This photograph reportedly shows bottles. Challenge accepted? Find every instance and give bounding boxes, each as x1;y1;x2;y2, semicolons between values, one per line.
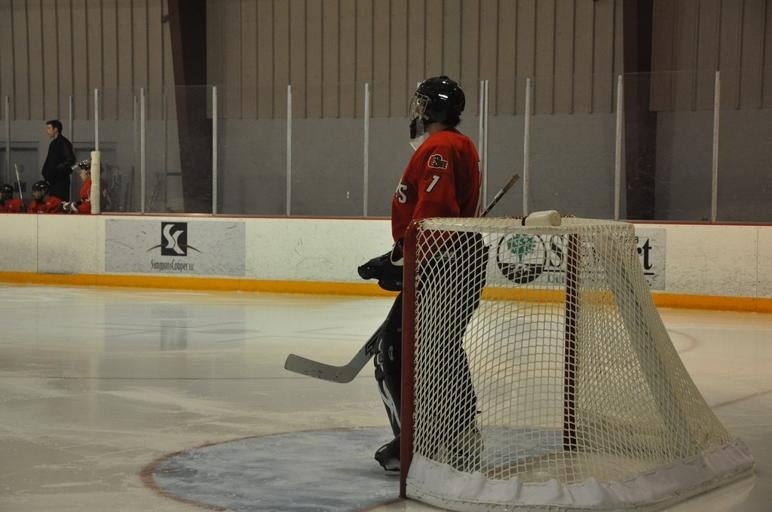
522;210;561;226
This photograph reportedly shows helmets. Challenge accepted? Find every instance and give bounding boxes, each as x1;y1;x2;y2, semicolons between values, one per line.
0;184;14;194
31;181;50;204
78;158;104;175
408;75;465;139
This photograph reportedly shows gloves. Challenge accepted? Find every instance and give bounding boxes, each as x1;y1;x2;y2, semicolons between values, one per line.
61;201;79;212
358;236;404;292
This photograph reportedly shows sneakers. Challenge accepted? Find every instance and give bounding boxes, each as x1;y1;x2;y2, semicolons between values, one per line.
374;430;485;470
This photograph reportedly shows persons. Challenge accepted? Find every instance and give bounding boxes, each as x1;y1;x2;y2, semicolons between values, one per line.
40;119;76;201
0;184;23;213
26;180;65;214
354;74;491;475
58;159;113;215
